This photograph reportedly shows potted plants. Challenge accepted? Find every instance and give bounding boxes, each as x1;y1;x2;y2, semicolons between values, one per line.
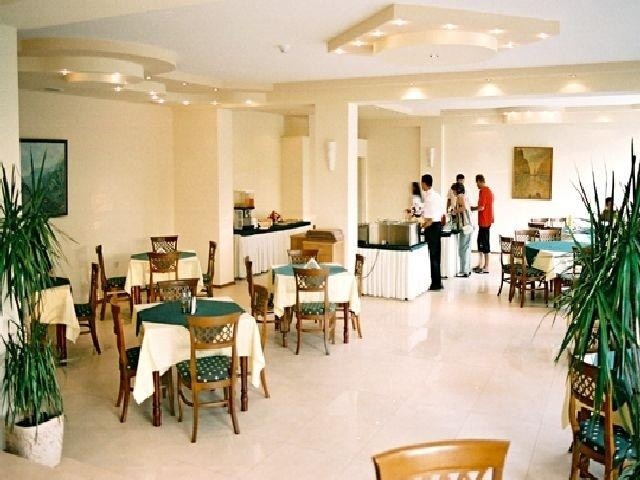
0;159;77;468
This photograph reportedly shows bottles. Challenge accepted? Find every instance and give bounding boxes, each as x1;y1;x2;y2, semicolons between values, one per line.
271;210;278;224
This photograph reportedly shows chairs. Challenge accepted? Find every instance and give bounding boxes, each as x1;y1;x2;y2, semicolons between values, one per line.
573;318;621;353
568;348;639;480
498;218;575;309
55;261;102;357
371;439;510;480
95;234;221;320
107;276;272;444
244;247;366;356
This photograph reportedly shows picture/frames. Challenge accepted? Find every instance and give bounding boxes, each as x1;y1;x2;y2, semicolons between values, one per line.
512;147;553;200
19;138;69;218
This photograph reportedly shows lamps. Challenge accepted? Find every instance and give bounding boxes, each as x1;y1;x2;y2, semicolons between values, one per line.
429;146;434;168
327;4;559;73
19;39;177;104
326;141;335;171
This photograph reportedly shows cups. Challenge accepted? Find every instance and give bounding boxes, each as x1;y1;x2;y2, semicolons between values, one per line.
180;289;197;316
251;217;256;229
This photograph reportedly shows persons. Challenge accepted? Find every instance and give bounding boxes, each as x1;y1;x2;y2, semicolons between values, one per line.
405;181;423;219
456;174;465;184
419;173;447;292
450;182;472;278
470;174;494;273
599;197;618;220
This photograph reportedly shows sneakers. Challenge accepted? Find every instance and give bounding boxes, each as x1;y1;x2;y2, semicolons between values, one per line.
454;265;489;278
